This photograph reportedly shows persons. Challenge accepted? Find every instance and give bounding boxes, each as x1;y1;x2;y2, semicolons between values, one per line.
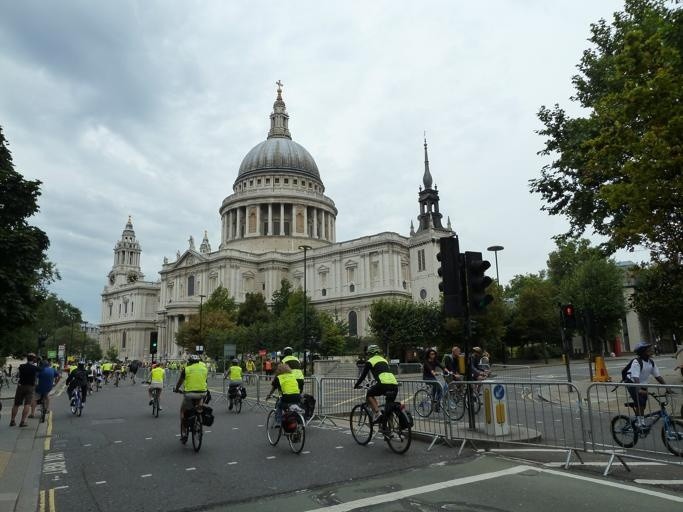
265;359;273;380
423;347;490;410
9;353;218;440
223;359;243;409
246;358;256;374
624;342;672;432
267;365;301;442
280;347;304;394
354;345;398;441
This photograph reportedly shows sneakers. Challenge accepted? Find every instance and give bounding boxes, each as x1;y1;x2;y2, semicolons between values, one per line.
635;420;648;431
371;410;383;424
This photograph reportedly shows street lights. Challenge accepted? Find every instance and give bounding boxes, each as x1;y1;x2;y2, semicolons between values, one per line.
198;294;207;343
297;244;313;376
53;300;61;347
81;320;88;332
68;312;79;353
308;334;317;361
487;245;504;287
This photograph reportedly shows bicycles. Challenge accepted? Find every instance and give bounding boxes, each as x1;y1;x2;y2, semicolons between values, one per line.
263;394;307;455
65;384;84;417
39;393;48;423
609;388;683;458
142;381;162;418
348;378;415;456
0;358;129;395
221;376;243;414
412;370;488;422
171;388;205;452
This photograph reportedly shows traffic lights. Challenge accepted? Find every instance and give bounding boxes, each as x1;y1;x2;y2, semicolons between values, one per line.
462;249;493;319
434;234;465;318
150;331;158;355
560;304;577;335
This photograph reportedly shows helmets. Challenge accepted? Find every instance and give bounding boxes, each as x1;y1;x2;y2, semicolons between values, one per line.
367;345;381;354
78;361;86;365
232;359;238;363
188;355;200;361
28;353;37;357
473;347;482;354
634;342;651;353
